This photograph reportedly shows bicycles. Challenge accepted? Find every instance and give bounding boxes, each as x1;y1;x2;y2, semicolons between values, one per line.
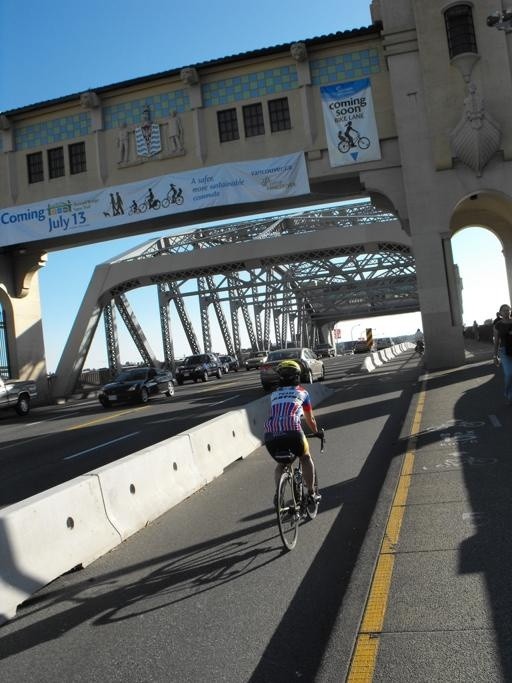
138;195;161;213
162;194;184;208
275;427;326;551
338;131;371;153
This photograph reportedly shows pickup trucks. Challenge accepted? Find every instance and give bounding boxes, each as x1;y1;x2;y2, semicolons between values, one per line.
0;374;38;416
313;343;335;358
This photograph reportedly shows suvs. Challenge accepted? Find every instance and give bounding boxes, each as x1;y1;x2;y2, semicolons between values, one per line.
175;353;222;385
376;336;394;350
354;340;375;353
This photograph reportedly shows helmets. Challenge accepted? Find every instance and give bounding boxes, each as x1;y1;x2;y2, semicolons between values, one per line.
276;360;300;375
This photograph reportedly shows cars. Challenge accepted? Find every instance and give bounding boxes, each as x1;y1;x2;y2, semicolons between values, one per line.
344;349;354;355
260;347;325;392
104;365;137;385
218;356;238;373
245;350;271;371
98;367;178;408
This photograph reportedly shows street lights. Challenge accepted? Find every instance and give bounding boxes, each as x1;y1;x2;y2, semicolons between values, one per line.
351;323;360;340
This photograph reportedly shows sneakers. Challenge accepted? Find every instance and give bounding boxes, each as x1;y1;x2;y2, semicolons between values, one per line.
307;494;322;503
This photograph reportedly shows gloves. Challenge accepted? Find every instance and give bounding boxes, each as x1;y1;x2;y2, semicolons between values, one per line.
316;428;325;437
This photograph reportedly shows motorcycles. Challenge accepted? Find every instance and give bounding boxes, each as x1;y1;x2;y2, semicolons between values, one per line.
416;339;424;355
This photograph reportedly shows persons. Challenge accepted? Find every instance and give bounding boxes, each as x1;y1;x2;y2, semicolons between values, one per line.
158;108;183;151
415;328;424;348
264;361;322;509
491;304;512;401
115;120;135;164
473;320;480;341
